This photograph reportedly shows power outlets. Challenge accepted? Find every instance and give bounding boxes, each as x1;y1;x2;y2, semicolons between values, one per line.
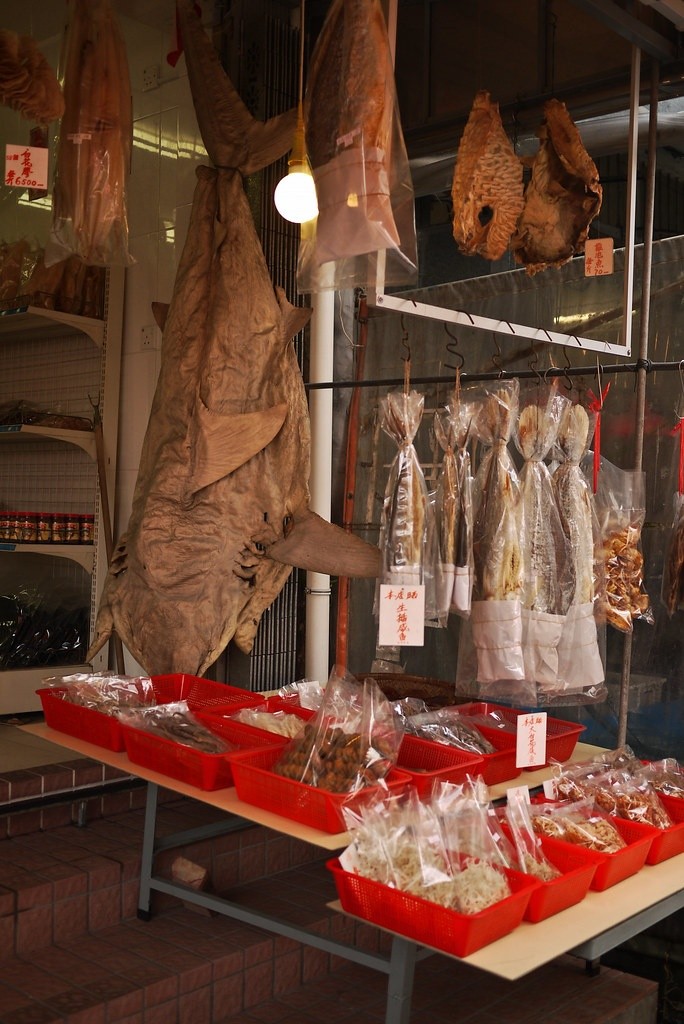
141;326;157;350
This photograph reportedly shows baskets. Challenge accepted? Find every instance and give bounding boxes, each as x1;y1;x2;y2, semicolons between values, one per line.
545;670;666;724
326;760;684;957
35;672;586;834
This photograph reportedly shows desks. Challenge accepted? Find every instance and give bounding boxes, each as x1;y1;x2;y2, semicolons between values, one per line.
16;690;684;1024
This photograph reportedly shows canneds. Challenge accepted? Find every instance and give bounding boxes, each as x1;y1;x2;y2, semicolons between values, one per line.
0;510;94;543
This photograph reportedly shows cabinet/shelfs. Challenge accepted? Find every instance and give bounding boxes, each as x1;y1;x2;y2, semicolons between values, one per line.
0;259;124;716
